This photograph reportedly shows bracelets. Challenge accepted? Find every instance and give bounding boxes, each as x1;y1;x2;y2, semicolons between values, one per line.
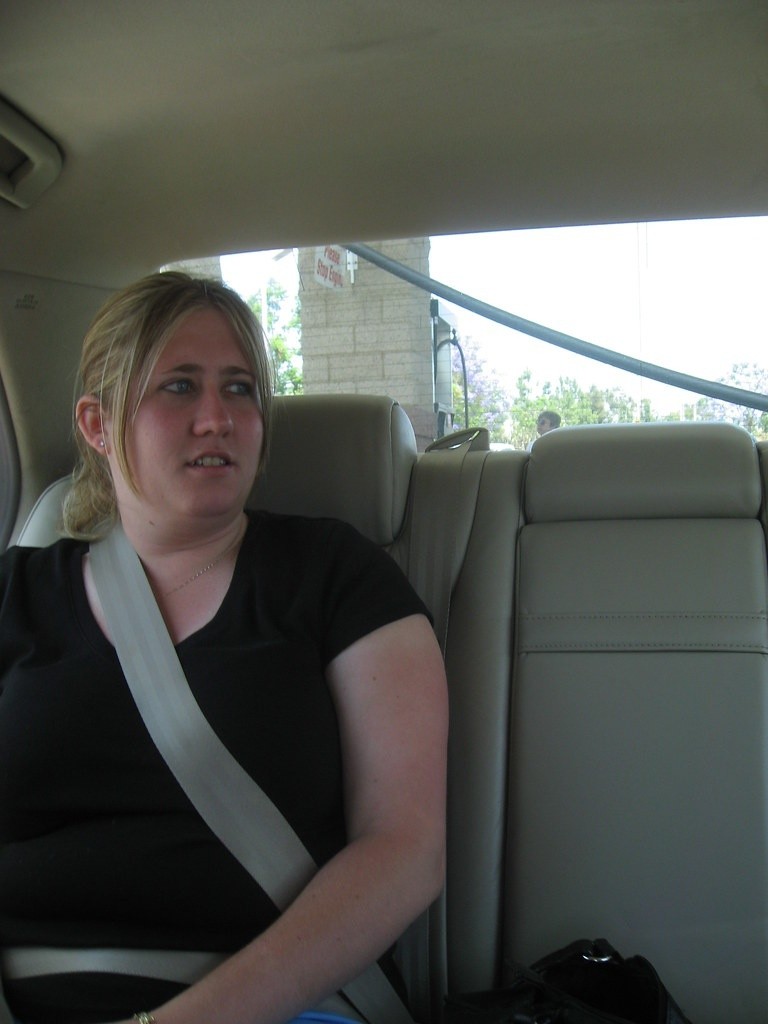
134;1012;158;1024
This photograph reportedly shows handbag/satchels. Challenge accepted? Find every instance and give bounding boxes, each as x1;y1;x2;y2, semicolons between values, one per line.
444;938;689;1024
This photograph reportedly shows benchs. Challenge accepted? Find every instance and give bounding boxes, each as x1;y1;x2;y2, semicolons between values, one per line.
8;388;768;1024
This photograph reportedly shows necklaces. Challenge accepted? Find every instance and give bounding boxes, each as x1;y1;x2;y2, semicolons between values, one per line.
157;521;243;599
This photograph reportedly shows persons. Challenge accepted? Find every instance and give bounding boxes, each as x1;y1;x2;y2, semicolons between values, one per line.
1;268;449;1024
537;411;560;435
